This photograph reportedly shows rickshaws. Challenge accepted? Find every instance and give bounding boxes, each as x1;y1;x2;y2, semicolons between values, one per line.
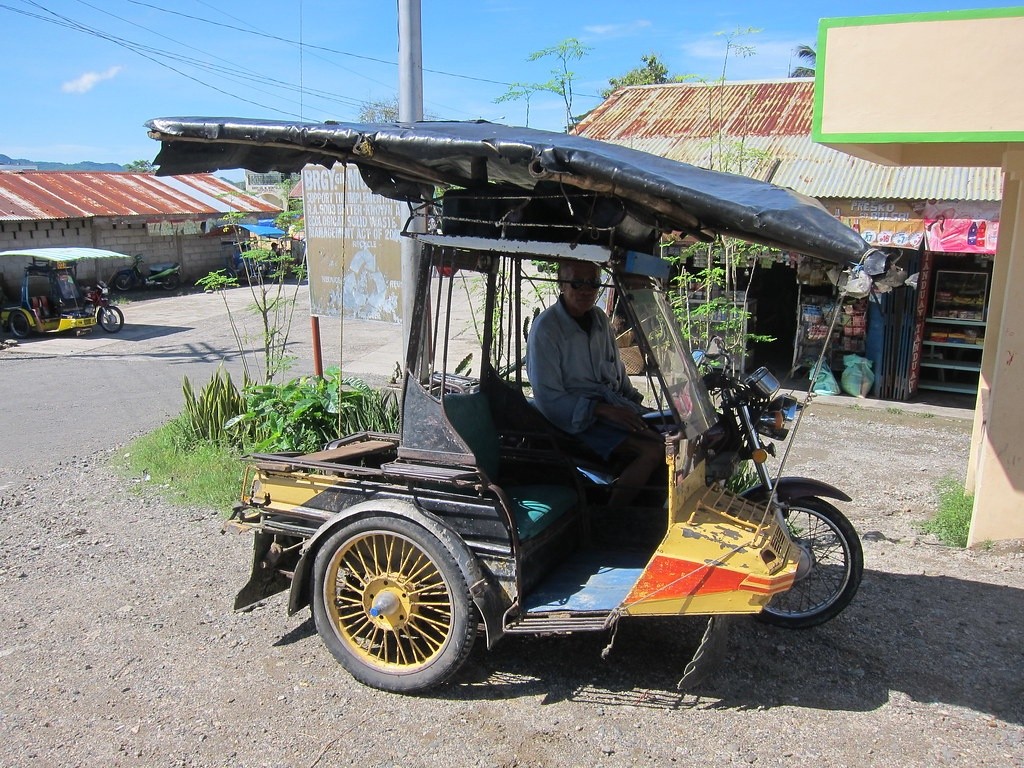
140;115;895;697
0;247;136;339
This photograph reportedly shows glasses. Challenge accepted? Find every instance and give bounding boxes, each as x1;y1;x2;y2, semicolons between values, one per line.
560;277;601;290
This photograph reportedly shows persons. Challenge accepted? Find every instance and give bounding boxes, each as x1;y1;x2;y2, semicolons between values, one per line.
269;243;280;264
234;245;251;276
526;257;666;503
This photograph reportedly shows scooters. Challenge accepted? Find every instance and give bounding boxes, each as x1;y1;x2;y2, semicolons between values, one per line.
112;254;183;292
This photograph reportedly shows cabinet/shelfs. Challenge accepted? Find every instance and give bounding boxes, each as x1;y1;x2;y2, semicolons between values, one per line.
917;319;987;396
689;296;758;374
932;270;989;322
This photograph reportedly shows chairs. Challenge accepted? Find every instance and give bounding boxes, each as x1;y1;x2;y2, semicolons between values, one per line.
417;392;596;584
29;295;54;319
480;355;677;517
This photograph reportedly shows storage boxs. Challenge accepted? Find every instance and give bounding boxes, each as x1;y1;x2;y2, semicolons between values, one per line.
844;311;868;337
